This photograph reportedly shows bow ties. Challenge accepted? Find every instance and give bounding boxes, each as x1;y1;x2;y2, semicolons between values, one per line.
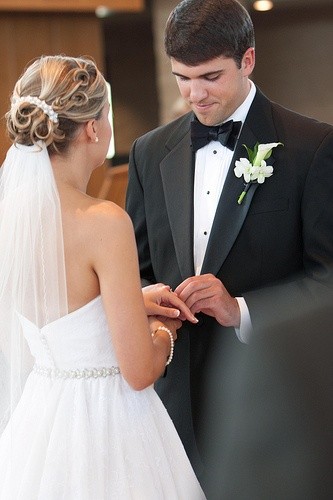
191;120;242;151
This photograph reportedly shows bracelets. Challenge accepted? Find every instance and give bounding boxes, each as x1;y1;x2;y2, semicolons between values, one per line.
152;326;174;367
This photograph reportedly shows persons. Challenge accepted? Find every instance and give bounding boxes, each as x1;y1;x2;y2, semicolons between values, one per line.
0;55;207;500
126;0;332;499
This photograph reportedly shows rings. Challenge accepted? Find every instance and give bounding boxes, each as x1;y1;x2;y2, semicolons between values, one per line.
170;287;172;293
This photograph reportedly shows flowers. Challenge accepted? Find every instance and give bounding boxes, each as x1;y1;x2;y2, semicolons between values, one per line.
234;142;284;205
